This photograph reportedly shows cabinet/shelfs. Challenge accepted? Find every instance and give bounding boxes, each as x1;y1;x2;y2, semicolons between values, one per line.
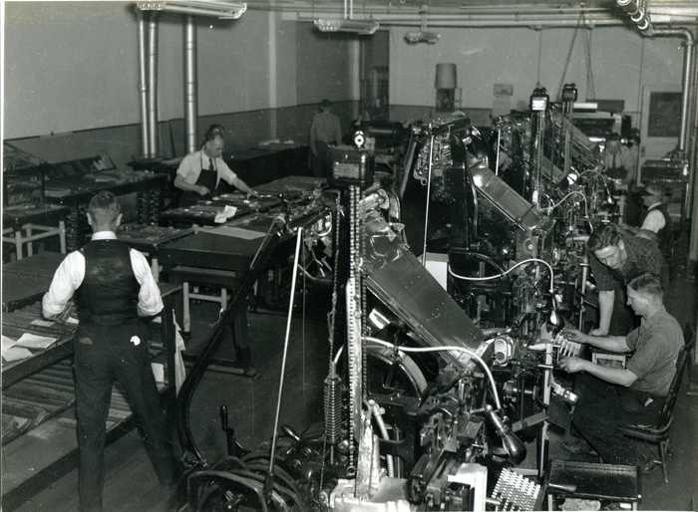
2;138;334;512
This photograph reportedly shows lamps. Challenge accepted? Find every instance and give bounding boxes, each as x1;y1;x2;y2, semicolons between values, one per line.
404;11;441;44
137;0;247;20
313;0;380;35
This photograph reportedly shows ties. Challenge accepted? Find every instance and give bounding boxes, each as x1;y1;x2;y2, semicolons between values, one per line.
209;159;213;169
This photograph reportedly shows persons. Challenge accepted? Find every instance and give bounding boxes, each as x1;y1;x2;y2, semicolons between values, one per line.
42;188;183;511
584;222;667;341
175;122;262;199
307;96;342;180
601;132;635;187
558;268;685;475
640;179;671;248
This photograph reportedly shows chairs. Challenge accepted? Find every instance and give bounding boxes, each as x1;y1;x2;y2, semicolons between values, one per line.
614;323;697;485
